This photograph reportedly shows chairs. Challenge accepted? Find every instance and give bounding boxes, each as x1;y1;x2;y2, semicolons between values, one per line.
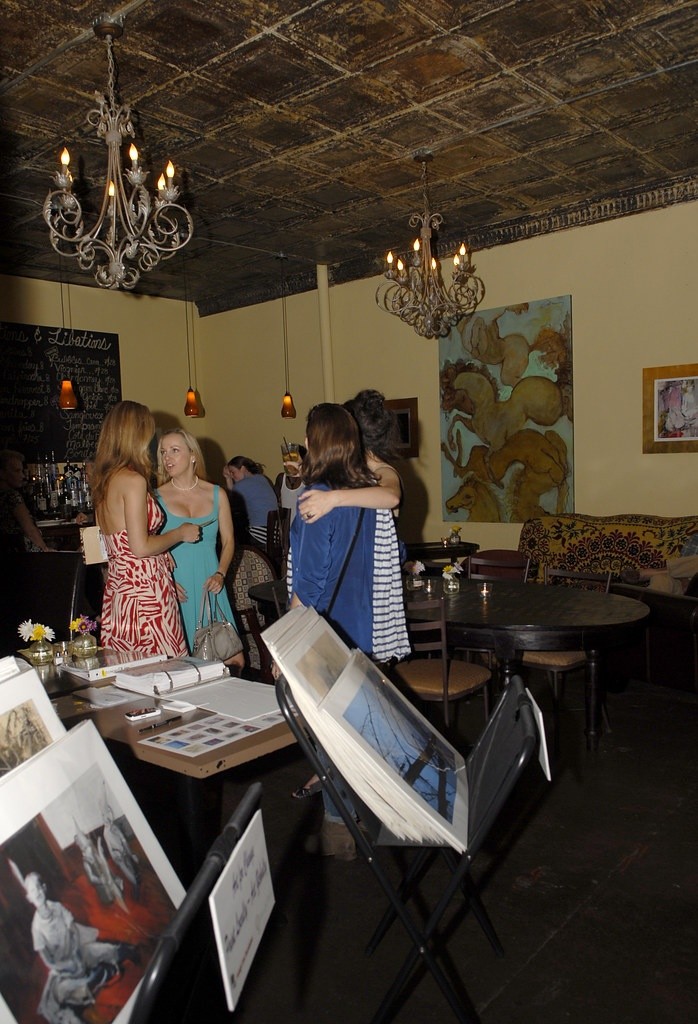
1;505;614;1024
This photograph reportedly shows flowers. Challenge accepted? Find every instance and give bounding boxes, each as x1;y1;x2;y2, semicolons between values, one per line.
68;613;103;633
15;616;59;646
403;560;425;575
441;562;464;579
450;524;462;535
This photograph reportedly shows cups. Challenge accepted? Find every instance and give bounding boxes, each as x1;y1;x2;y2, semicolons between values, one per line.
441;537;450;545
281;443;298;476
422;579;437;593
478;582;494;598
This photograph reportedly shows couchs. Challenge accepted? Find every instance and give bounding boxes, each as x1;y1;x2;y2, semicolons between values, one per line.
519;511;698;684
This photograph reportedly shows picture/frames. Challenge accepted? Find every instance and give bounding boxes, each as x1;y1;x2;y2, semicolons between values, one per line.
643;366;698;454
379;398;418;460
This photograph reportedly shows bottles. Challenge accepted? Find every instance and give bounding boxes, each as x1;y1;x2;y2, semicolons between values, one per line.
22;450;96;520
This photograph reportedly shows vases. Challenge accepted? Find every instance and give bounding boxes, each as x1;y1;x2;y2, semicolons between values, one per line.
443;574;459;594
29;639;54;665
68;633;103;658
406;574;423;590
449;534;459;544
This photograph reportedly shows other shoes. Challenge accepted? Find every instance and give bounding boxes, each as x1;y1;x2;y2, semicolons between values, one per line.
290;780;322;799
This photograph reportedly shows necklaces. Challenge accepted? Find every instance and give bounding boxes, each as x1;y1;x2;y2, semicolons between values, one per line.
171;474;198;491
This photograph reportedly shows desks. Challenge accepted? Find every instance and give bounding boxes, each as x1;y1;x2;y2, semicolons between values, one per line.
245;576;652;761
405;542;479;578
2;644;302;885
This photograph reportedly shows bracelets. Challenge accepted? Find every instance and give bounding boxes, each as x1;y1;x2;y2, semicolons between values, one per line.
216;571;224;579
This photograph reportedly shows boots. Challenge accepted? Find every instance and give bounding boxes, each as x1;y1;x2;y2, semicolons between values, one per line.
300;818;369;861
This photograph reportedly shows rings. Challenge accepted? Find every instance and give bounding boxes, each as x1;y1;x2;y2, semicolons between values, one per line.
307;513;311;518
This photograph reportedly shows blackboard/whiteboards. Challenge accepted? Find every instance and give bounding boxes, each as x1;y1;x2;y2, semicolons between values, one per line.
0;321;122;463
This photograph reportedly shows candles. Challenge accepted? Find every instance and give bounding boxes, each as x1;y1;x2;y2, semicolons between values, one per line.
443;541;447;545
426;585;432;593
481;590;490;595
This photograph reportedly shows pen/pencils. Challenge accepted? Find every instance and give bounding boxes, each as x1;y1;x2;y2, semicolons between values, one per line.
138;715;182;733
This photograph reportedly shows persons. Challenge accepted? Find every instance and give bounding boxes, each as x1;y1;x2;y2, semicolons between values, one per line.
0;450;59;552
90;388;418;859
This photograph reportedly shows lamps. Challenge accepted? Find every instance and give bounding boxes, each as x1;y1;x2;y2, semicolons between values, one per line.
373;149;486;338
55;232;76;413
42;12;192;291
277;252;298;421
172;232;205;419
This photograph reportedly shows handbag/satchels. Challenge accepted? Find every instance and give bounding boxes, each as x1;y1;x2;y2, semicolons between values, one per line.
192;584;243;662
